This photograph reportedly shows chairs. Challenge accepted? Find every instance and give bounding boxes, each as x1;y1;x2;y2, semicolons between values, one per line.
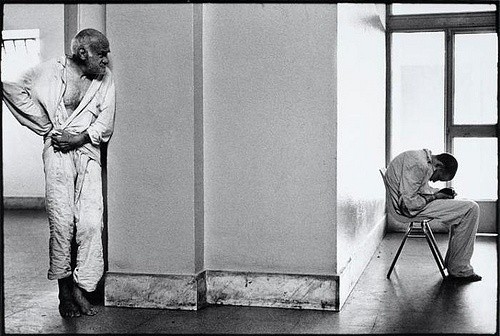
380;166;450;279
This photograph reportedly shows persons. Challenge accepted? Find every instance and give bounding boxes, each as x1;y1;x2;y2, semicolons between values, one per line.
0;29;116;317
385;149;482;281
